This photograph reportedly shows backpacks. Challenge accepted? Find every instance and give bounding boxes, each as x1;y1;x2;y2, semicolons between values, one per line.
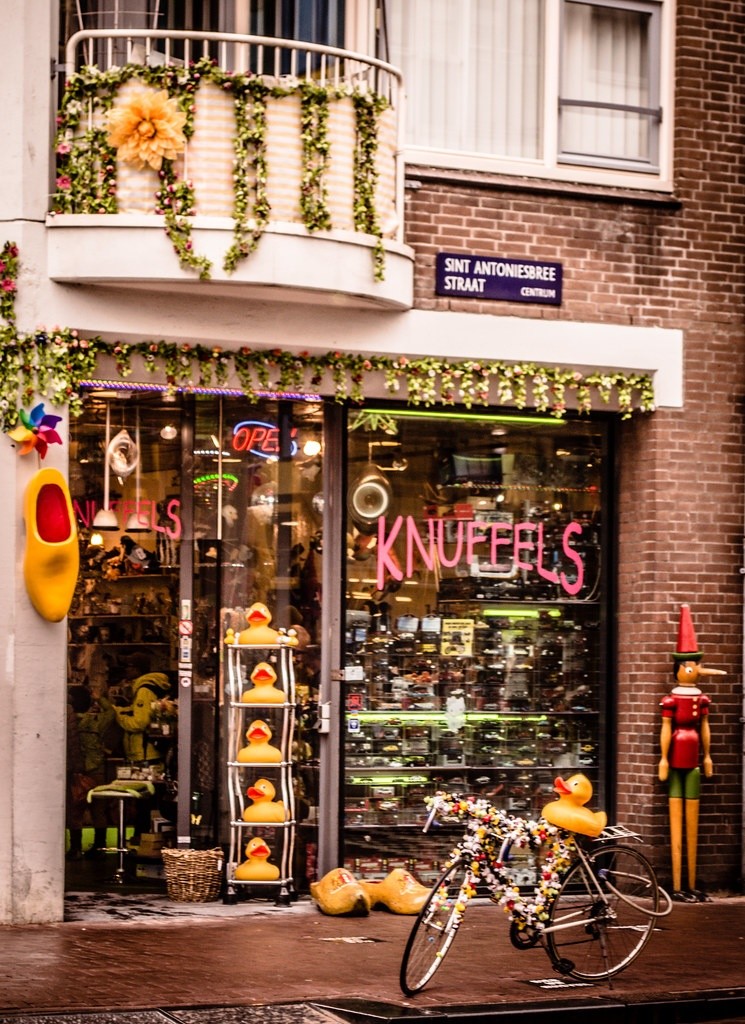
132;684;176;755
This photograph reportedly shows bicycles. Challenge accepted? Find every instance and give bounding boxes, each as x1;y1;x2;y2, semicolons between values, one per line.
399;795;663;996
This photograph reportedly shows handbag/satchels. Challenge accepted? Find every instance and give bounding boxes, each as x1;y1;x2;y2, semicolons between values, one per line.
67;768;96;804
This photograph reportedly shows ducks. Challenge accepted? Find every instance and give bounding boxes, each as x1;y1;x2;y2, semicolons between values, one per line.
541;772;607;837
235;601;291;882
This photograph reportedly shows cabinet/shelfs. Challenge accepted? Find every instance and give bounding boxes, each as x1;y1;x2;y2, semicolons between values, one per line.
227;627;301;908
64;574;171;647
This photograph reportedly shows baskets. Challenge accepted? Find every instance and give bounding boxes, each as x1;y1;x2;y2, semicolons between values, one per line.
160;847;223;903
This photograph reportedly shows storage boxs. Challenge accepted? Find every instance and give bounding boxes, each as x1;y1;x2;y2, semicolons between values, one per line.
421;496;602;600
303;617;599;886
118;765;174;857
136;863;167;880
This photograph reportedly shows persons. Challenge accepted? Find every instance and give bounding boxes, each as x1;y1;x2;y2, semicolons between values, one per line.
66;685;118;860
114;652;177;846
658;603;729;903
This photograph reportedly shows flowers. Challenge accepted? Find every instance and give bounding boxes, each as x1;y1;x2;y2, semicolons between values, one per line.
104;77;189;172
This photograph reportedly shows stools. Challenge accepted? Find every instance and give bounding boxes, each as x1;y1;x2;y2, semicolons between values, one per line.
91;790;147;884
